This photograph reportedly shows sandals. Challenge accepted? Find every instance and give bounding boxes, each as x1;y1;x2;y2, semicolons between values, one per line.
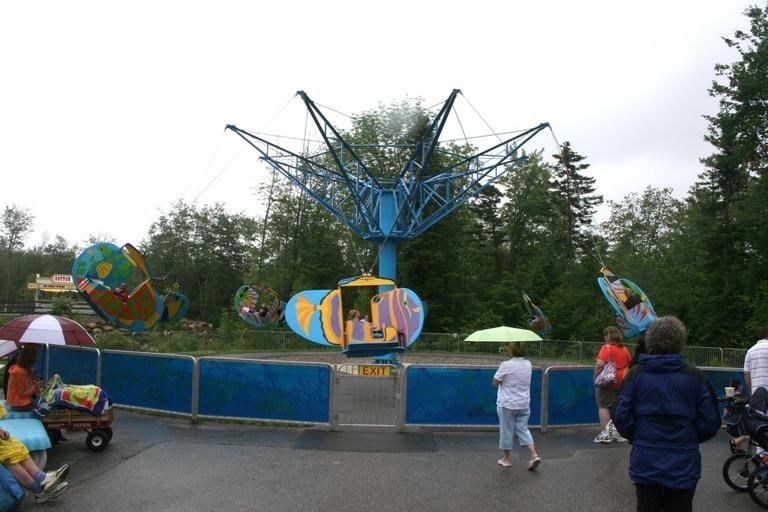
728;439;737;455
734;447;747;454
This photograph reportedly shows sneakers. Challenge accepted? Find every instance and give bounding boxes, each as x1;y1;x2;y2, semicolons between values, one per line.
32;482;68;504
41;464;71;493
526;456;543;472
593;434;612;444
498;457;512;469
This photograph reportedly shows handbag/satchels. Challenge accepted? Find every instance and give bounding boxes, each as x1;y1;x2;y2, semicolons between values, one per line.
592;360;618;387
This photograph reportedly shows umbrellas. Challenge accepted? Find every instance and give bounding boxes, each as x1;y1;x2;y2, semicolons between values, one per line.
464;326;544;362
0;314;96;388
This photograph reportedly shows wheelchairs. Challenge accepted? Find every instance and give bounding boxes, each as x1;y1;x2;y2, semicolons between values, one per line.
717;388;768;512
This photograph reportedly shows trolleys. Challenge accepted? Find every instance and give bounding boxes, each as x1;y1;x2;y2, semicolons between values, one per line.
36;383;113;451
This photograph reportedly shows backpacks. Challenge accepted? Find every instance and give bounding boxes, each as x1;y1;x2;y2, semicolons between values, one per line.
0;461;26;512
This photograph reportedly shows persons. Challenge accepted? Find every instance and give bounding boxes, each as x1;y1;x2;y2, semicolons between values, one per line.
0;403;69;504
244;303;269;321
623;287;642;310
743;326;768;459
347;308;361;321
592;326;632;443
493;343;541;471
722;377;751;454
361;314;369;321
114;282;132;305
7;349;64;445
3;348;69;447
612;315;722;511
